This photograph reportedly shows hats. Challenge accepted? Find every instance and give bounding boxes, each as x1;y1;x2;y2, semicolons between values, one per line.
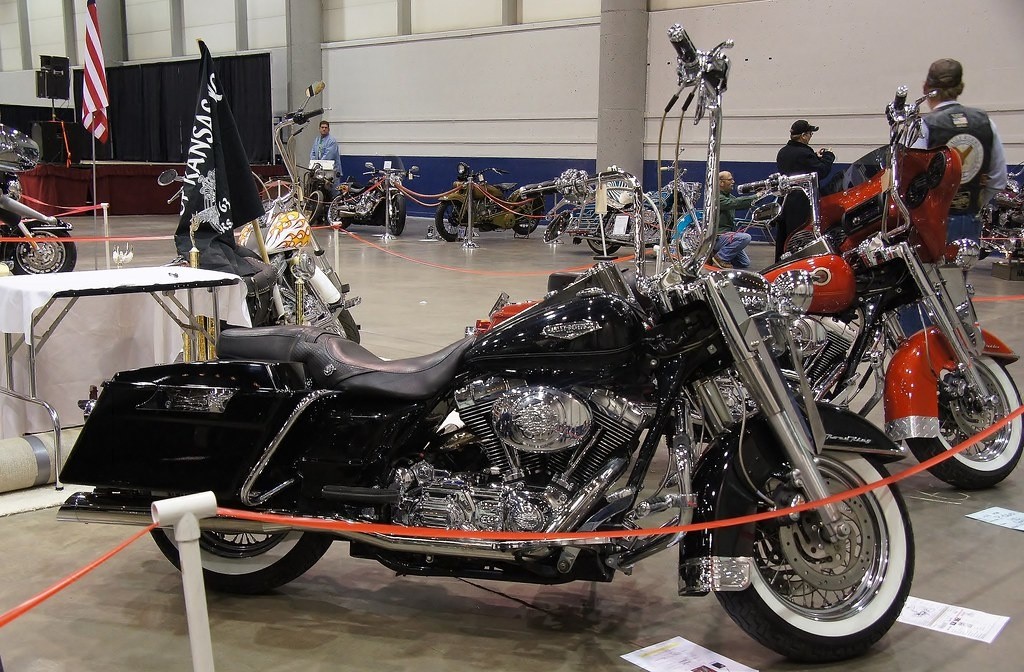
789;120;820;136
928;58;963;88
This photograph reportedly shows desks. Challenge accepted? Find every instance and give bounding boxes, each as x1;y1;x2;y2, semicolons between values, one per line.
0;265;240;490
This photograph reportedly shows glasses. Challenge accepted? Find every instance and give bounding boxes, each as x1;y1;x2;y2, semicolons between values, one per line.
720;178;734;181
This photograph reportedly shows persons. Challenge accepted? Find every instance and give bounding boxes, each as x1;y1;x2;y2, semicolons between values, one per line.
775;120;836;263
897;57;1008;338
310;121;343;226
711;170;765;269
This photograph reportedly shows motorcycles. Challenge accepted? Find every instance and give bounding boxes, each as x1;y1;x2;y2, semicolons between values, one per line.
296;160;1023;278
54;25;918;666
156;79;362;344
465;83;1024;492
0;123;76;277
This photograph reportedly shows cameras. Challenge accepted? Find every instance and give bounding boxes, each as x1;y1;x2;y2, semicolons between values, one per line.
820;149;828;155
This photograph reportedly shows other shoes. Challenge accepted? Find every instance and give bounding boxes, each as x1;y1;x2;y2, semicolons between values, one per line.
705;250;716;266
713;253;733;269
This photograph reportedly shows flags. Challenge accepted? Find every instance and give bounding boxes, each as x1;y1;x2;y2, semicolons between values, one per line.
174;37;266;277
82;0;110;144
